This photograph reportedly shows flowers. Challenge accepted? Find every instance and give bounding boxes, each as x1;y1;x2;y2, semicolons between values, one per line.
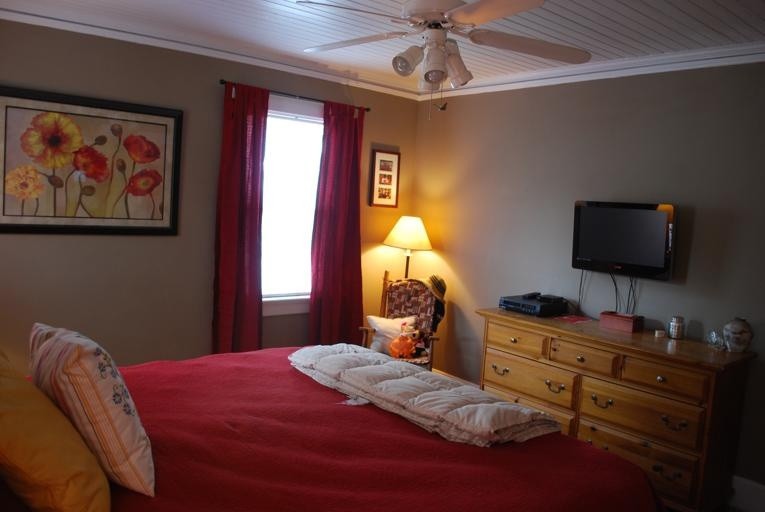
4;106;165;219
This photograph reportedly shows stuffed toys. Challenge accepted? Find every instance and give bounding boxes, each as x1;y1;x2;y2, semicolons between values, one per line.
388;320;427;359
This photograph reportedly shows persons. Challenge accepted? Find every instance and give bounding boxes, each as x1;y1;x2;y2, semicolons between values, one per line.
378;160;392;199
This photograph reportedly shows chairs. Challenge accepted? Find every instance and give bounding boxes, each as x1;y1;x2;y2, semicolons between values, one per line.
357;271;446;371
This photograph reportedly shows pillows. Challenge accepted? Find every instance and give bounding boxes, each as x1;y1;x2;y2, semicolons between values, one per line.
27;322;162;499
0;365;111;512
362;314;417;352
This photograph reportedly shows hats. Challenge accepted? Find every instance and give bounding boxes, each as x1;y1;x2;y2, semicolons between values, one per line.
420;274;447;303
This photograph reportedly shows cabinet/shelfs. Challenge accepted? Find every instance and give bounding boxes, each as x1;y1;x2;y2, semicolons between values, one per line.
471;298;753;509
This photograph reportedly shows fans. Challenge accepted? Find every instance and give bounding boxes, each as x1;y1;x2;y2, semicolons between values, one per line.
283;0;593;94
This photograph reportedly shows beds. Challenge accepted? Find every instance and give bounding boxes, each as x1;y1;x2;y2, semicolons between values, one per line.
0;345;665;511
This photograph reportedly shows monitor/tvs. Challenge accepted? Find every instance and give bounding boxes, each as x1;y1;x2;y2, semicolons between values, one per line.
570;198;677;286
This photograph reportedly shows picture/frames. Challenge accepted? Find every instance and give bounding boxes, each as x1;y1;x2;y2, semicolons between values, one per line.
0;84;187;240
369;148;401;207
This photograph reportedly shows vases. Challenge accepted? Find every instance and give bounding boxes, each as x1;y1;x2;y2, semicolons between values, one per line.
720;314;752;355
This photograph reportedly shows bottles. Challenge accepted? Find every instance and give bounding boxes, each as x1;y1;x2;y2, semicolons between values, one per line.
669;316;685;339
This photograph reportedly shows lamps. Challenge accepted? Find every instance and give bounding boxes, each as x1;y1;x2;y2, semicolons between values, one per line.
386;32;473;116
377;215;433;279
